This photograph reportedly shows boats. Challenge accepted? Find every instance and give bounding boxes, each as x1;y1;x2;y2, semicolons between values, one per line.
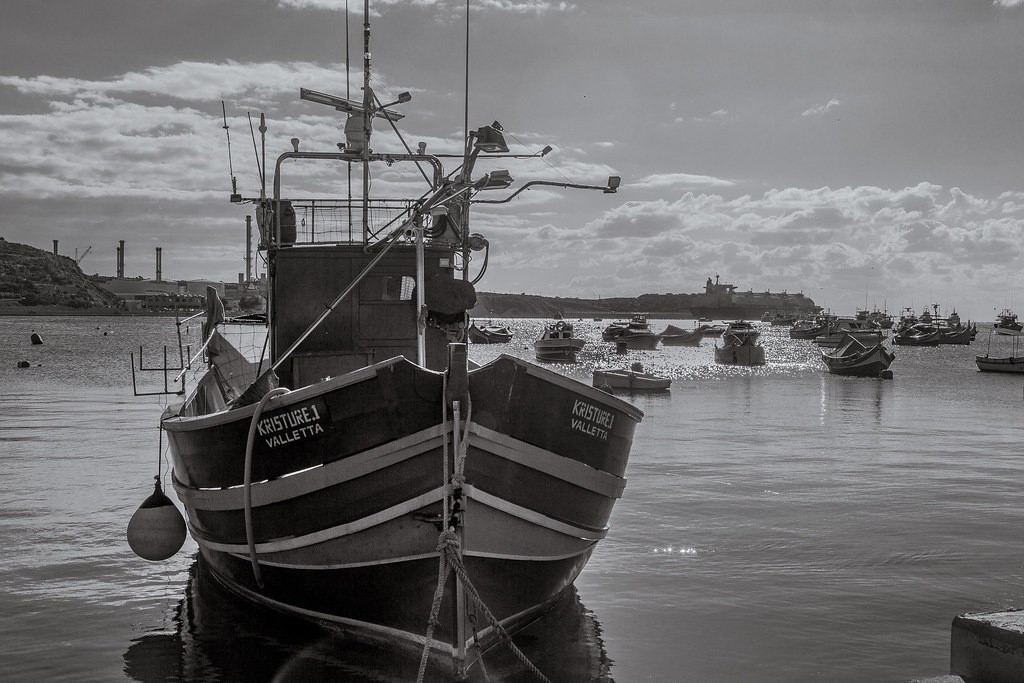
125;1;645;678
976;330;1024;374
721;322;761;348
757;302;1024;348
657;324;707;345
818;334;896;374
697;320;730;336
713;330;766;366
590;363;671;391
616;314;661;351
123;557;613;683
